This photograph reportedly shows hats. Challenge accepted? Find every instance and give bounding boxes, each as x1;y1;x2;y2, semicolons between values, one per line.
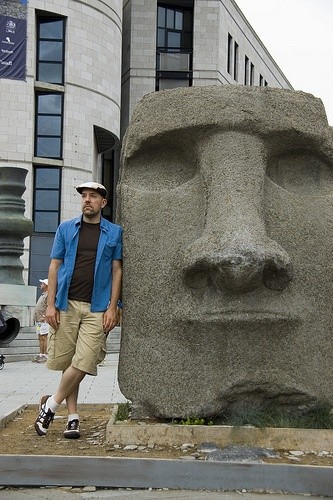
75;182;107;199
38;278;48;285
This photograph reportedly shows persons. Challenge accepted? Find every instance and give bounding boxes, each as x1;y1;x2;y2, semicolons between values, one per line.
33;279;48;363
116;84;331;424
116;293;122;326
34;182;123;438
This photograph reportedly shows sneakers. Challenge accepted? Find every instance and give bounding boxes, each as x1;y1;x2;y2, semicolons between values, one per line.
63;418;81;439
34;394;56;436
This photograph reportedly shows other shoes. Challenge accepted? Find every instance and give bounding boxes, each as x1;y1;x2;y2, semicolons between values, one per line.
31;354;40;362
37;354;47;363
